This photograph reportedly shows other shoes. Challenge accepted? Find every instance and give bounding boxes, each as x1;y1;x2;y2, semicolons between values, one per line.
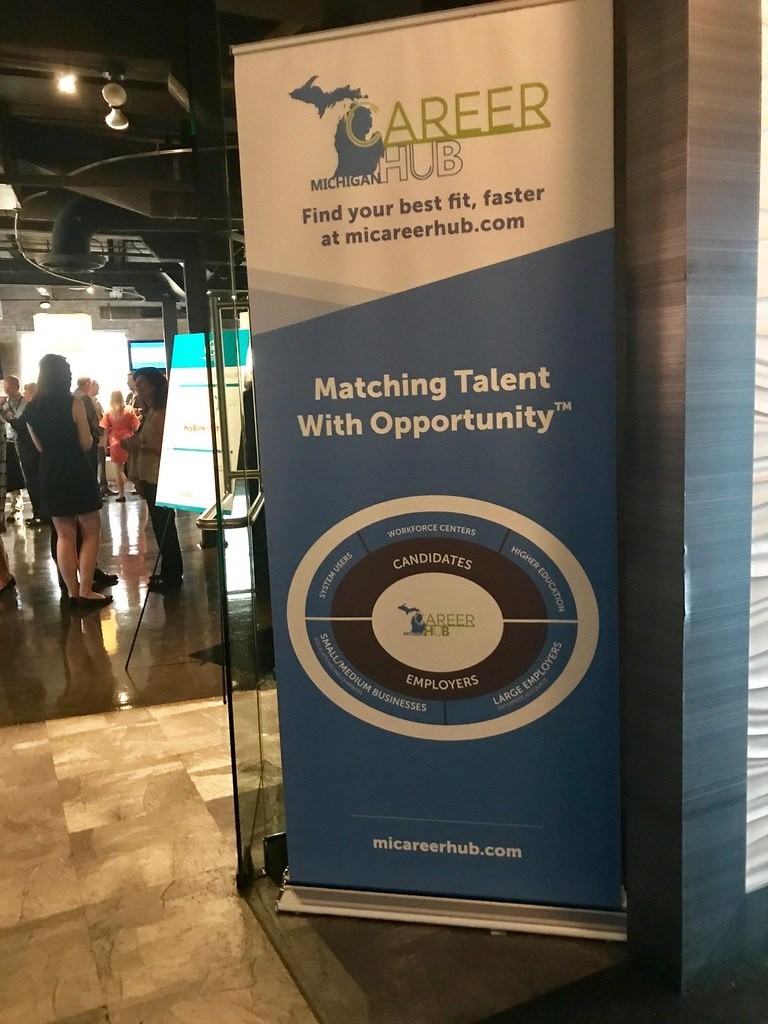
116;497;125;502
148;578;183;589
25;514;50;525
93;569;119;582
0;574;16;596
149;573;164;580
68;597;78;608
6;516;15;522
99;485;118;496
78;592;112;608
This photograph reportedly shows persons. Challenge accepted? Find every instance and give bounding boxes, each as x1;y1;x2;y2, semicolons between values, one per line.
0;354;148;608
120;367;184;589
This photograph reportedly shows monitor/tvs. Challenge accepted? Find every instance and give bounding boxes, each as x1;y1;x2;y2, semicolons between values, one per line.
127;339;166;372
